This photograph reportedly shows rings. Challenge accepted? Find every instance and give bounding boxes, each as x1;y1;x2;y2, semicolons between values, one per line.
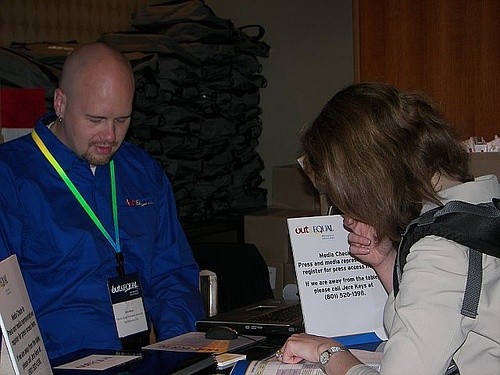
275;350;283;358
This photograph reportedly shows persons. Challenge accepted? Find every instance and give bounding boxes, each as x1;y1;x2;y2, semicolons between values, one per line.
275;82;500;375
0;42;208;361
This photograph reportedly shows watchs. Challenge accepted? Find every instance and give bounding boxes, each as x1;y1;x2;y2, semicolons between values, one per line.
319;347;351;369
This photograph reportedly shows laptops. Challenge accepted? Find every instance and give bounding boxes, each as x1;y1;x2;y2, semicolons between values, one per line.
195;299;305;334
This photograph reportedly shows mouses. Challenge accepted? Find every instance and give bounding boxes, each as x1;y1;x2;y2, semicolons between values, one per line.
205;326;239;340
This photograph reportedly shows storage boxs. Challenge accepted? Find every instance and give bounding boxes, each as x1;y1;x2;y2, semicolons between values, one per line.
244;165;320;300
